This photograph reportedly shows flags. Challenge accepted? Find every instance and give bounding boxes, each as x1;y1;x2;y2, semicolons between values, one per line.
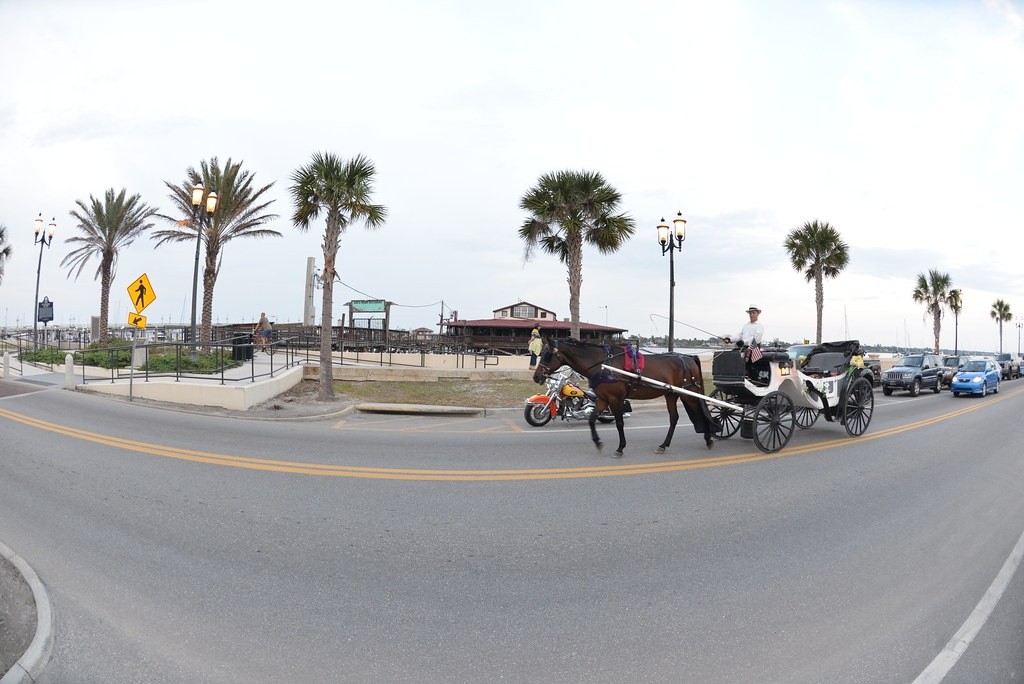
748;339;763;364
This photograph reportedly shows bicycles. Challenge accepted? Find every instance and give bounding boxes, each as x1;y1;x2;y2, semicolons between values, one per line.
250;329;278;356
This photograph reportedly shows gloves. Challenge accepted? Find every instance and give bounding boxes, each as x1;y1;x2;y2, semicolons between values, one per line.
723;337;730;344
736;340;744;347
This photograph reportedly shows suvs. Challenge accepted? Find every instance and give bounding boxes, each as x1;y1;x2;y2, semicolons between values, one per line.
783;341;819;368
942;354;1024;390
881;353;945;398
995;353;1020;379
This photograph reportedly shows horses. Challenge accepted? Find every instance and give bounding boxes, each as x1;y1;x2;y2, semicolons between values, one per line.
533;334;723;460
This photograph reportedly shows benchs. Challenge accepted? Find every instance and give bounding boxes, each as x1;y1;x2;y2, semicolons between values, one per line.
802;353;847;377
732;349;789;363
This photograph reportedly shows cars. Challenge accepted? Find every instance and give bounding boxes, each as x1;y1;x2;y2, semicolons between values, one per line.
950;359;1002;398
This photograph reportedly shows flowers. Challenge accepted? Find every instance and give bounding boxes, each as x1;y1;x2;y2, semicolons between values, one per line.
848;355;864;377
799;355;807;371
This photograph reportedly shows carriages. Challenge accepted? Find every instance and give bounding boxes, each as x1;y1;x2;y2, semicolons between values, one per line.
533;334;875;459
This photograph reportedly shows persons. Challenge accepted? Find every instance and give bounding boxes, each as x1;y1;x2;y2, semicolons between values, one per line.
741;302;765;382
527;323;542;370
254;312;272;349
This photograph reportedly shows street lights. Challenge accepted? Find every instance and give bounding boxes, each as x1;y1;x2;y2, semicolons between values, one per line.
1015;316;1024;356
188;181;218;359
31;212;56;350
657;210;688;353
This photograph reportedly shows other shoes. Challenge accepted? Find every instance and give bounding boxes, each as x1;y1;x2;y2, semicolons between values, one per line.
529;366;536;369
262;348;266;352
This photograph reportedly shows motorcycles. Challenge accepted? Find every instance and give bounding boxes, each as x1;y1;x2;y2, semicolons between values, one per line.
524;365;633;428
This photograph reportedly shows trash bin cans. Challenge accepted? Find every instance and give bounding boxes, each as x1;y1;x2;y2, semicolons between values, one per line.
134;337;149;367
232;331;255;361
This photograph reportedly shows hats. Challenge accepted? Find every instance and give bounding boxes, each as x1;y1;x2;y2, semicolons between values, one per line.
534;323;541;328
746;304;761;315
